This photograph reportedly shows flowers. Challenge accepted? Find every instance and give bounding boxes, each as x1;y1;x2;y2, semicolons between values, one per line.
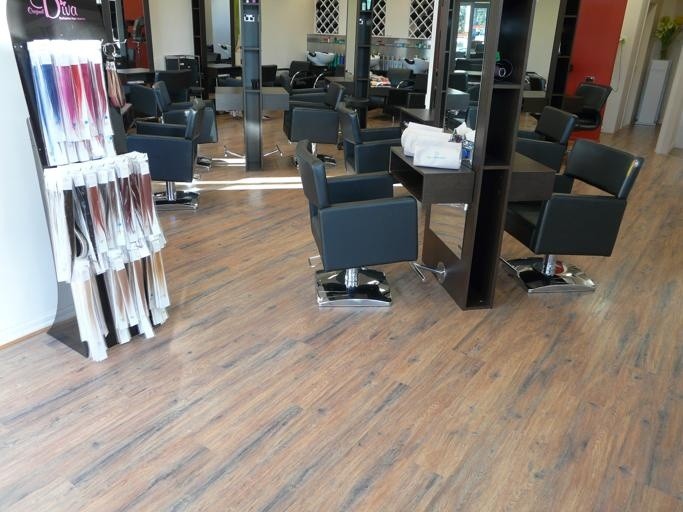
653;11;682;59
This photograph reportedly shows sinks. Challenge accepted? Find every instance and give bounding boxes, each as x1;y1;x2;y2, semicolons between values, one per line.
215;43;231;58
370;54;380;69
400;57;429;74
305;50;336;67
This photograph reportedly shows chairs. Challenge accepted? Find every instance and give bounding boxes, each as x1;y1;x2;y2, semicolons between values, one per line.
295;139;418;306
119;52;218;171
499;138;644;294
516;105;578;172
282;82;346;167
523;71;545;91
263;51;427;128
530;81;613;156
121;98;205;211
338;106;407;175
440;41;485;132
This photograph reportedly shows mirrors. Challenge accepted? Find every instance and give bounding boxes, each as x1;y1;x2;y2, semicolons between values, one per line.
426;0;488;260
121;0;148;67
344;0;357;80
204;0;232;64
